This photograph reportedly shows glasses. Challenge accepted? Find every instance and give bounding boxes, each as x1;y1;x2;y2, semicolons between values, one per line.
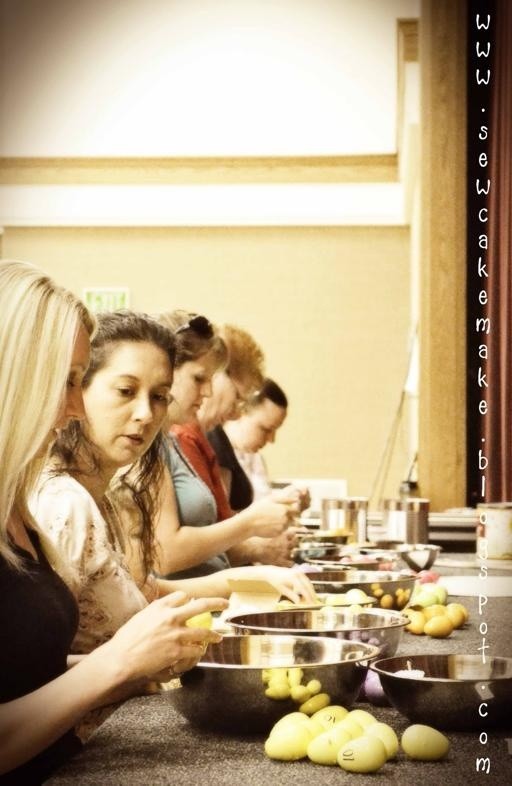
173;315;214;340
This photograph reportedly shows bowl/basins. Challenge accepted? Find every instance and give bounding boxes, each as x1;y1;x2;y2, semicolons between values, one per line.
159;540;512;735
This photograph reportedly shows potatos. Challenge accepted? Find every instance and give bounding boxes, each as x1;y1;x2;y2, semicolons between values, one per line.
186;613;213;631
264;706;447;773
399;603;469;637
259;669;329;714
370;584;411;609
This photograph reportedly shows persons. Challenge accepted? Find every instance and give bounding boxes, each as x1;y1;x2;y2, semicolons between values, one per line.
111;308;312;580
170;323;266;520
16;304;318;697
220;380;290;502
0;259;233;786
